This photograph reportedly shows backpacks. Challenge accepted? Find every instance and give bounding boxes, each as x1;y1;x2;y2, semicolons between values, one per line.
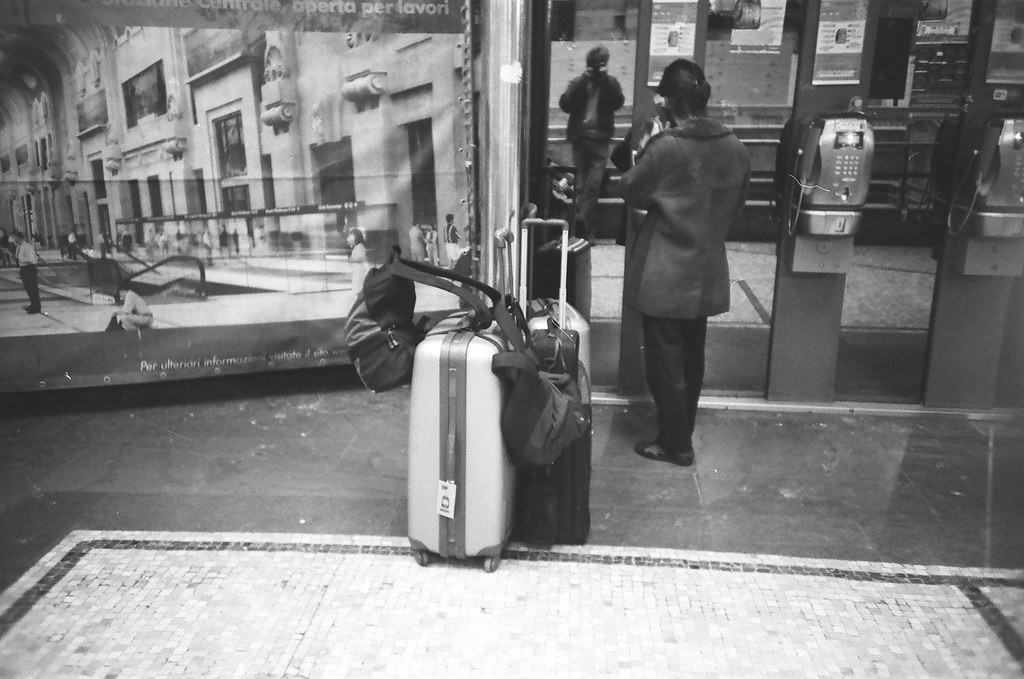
492;295;590;547
344;245;499;395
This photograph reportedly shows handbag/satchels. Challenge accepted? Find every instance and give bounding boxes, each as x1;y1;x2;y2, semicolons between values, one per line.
106;317;124;331
611;142;630;172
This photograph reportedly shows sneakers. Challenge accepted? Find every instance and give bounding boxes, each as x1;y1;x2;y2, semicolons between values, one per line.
636;441;694;466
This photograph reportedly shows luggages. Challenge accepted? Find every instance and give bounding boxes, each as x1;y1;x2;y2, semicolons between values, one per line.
508;218;590;406
409;309;515;572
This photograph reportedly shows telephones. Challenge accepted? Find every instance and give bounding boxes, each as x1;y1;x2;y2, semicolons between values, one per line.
976;118;1023;213
794;108;875;210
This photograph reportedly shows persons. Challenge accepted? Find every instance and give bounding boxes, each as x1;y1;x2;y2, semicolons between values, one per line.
58;226;237;264
619;58;751;467
0;228;42;314
422;223;440;266
559;45;626;249
346;228;369;293
111;285;155;332
409;219;430;262
443;213;460;268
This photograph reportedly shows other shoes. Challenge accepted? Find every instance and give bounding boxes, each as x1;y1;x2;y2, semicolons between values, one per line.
28;309;40;314
25;307;30;311
616;238;625;246
